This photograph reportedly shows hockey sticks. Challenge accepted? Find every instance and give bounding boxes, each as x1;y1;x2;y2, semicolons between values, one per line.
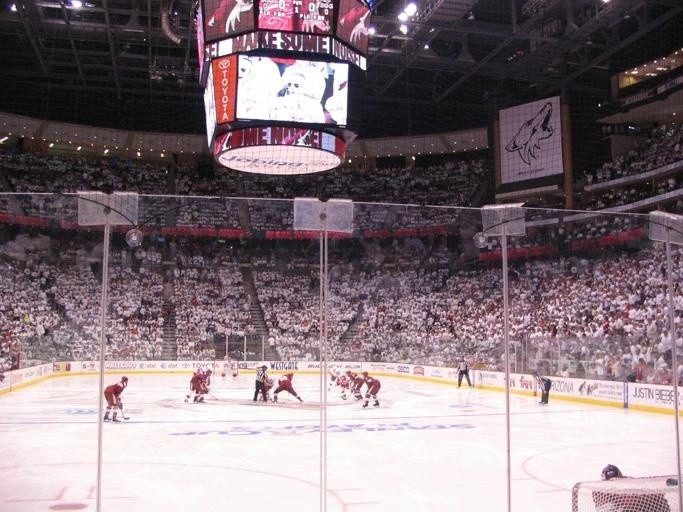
287;391;292;401
343;386;356;400
118;399;130;420
207;390;218;400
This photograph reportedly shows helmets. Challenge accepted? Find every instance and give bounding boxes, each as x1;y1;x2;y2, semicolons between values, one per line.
362;372;368;377
601;463;622;480
345;370;350;376
120;376;128;384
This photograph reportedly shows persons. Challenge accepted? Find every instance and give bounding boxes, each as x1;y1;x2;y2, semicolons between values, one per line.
592;465;671;512
236;55;349;125
104;376;128;422
0;123;683;408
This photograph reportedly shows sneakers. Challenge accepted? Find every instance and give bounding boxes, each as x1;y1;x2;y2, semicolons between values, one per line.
372;400;379;406
272;396;277;404
184;394;190;403
362;401;368;407
103;415;121;422
193;396;205;403
296;397;302;401
354;395;362;400
